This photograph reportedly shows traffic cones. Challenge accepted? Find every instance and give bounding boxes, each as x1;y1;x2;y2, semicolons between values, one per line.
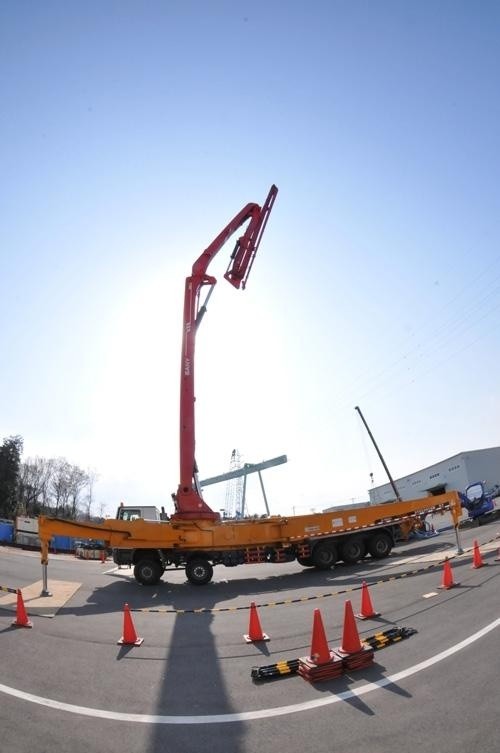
352;580;381;622
242;601;271;646
470;538;489;570
295;599;374;683
116;602;145;648
436;554;461;590
11;588;34;628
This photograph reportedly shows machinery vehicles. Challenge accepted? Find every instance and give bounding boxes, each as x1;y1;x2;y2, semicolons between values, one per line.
457;478;500;529
354;405;440;543
36;182;466;599
